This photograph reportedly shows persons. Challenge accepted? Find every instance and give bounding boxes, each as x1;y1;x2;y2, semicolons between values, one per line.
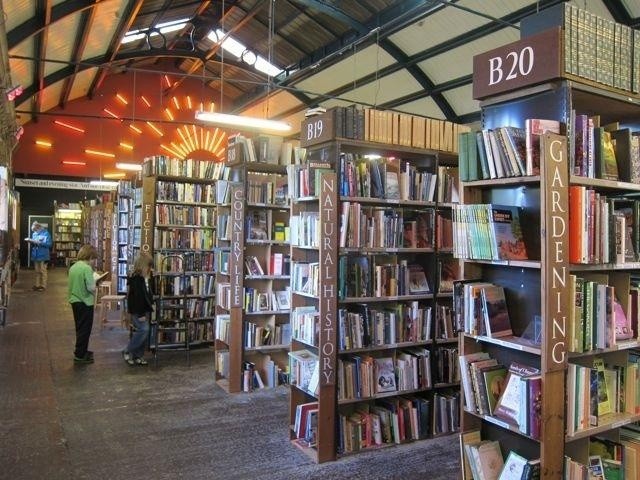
122;256;154;365
65;243;99;365
24;220;55;294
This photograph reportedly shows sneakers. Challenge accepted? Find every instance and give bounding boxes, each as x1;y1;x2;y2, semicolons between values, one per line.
122;350;135;367
134;356;148;365
73;357;94;364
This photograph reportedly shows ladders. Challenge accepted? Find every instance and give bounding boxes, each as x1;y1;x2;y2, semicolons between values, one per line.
154;256;191;368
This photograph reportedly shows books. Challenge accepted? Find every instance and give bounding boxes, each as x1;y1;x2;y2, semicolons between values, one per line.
112;172;143;308
287;161;332;447
336;152;461;453
564;113;639;480
53;193;111;286
229;134;307;391
24;238;35;243
452;119;560;480
143;155;234;379
0;244;21;331
